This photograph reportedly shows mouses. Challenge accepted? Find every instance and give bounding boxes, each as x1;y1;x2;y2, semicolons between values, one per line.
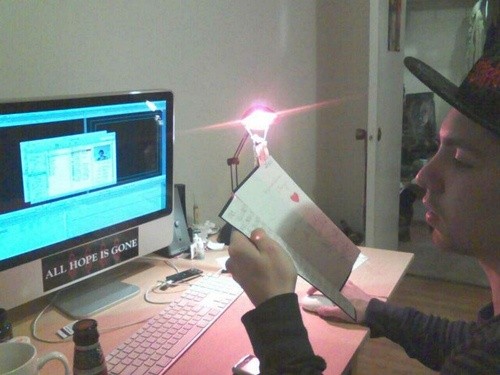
302;292;335;313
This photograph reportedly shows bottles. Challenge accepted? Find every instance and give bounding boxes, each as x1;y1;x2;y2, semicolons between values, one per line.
72;318;110;375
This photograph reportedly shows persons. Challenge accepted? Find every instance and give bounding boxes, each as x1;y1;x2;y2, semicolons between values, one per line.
224;28;499;375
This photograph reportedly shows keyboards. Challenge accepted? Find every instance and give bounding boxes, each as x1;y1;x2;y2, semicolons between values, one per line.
105;272;245;375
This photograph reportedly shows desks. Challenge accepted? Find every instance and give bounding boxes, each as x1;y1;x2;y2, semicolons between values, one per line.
8;236;414;375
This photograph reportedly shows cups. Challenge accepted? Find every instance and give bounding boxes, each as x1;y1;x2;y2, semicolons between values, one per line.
0;341;70;375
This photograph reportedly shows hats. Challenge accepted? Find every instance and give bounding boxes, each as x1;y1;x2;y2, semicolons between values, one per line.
403;55;500;135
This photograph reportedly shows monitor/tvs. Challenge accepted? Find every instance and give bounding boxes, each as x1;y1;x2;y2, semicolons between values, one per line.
1;88;177;321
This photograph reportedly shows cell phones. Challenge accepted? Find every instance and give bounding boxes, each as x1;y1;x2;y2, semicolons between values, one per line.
232;354;261;375
167;268;203;285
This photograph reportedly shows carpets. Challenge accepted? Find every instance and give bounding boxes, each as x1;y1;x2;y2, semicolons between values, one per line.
401;234;490;288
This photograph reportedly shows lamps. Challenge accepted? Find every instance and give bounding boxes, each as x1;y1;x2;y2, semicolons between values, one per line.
215;103;276;243
